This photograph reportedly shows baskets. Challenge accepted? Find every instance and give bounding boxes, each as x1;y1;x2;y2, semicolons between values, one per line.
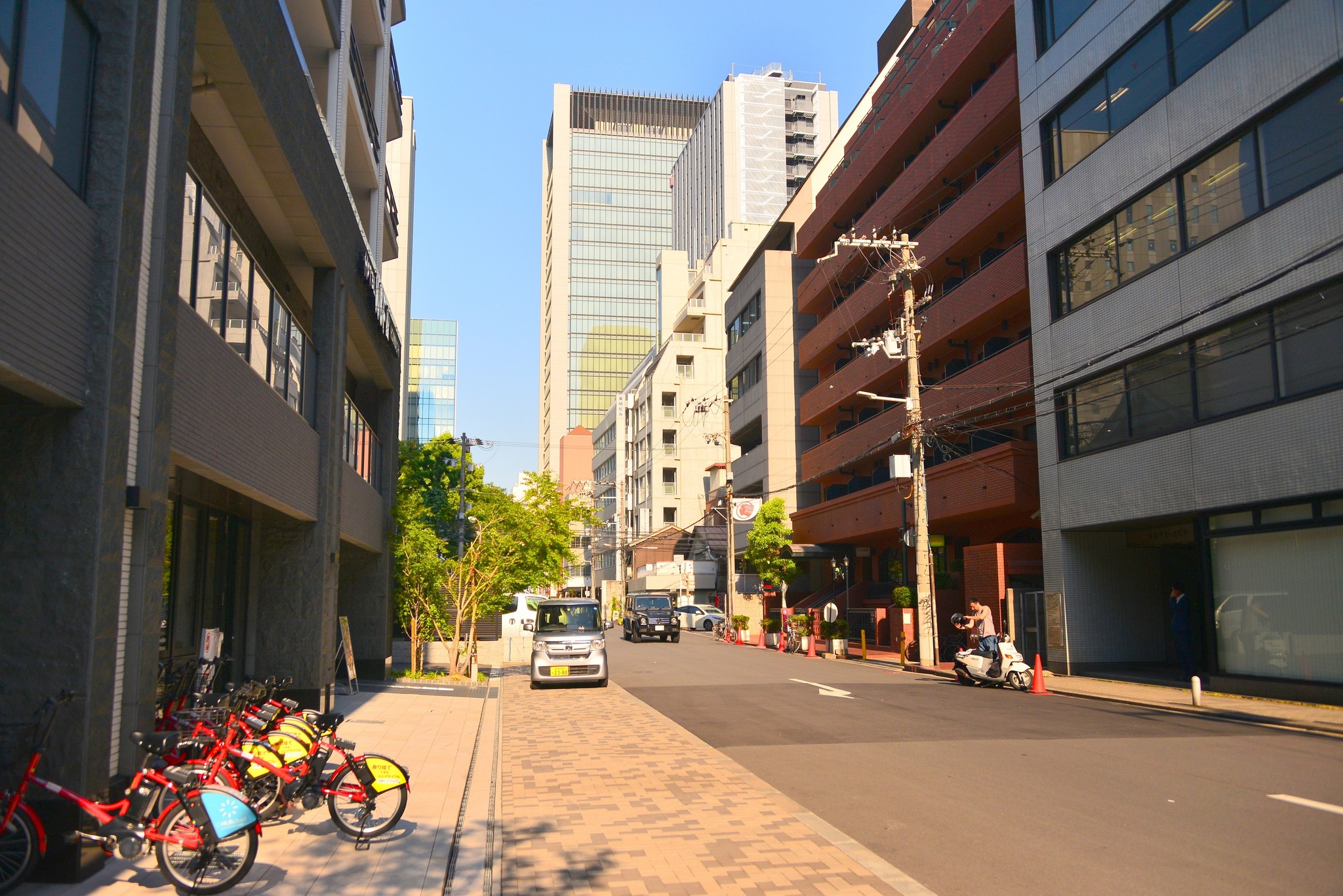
728;619;736;627
795;625;804;634
155;670;229;746
0;722;43;769
948;634;959;644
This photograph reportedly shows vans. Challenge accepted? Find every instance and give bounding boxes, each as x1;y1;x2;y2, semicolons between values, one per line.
502;592;567;637
1215;591;1290;641
524;598;614;690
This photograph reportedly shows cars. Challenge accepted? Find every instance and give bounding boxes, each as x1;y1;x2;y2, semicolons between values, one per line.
674;604;726;631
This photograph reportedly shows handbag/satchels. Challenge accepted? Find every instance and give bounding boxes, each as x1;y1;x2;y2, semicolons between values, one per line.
988;659;1001;678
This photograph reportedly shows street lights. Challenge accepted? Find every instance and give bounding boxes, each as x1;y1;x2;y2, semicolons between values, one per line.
857;389;912;411
831;555;850;641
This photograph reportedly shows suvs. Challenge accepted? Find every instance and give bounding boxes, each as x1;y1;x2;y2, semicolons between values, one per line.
622;593;681;643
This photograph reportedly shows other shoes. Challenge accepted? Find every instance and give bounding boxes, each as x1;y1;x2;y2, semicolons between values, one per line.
1176;674;1193;682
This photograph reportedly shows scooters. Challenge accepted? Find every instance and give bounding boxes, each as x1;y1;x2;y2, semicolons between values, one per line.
952;620;1034;690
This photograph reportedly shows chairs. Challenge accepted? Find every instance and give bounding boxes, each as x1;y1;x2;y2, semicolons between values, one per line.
545;613;564;626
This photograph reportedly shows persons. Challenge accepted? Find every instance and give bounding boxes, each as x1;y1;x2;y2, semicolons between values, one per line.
637;599;648;608
1169;583;1197;682
955;597;1004;688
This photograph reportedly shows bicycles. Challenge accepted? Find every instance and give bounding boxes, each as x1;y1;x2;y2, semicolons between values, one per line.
1;655;412;895
785;618;804;654
905;628;966;662
712;614;737;643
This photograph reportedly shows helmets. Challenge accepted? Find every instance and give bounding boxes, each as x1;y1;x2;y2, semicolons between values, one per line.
951;613;966;626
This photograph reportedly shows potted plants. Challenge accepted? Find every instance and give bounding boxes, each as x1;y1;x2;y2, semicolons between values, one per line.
610;597;623;625
731;615;750;641
788;615;816;651
818;619;852;655
758;617;783;646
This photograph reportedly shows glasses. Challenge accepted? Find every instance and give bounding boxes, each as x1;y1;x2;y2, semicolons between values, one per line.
969;604;976;609
